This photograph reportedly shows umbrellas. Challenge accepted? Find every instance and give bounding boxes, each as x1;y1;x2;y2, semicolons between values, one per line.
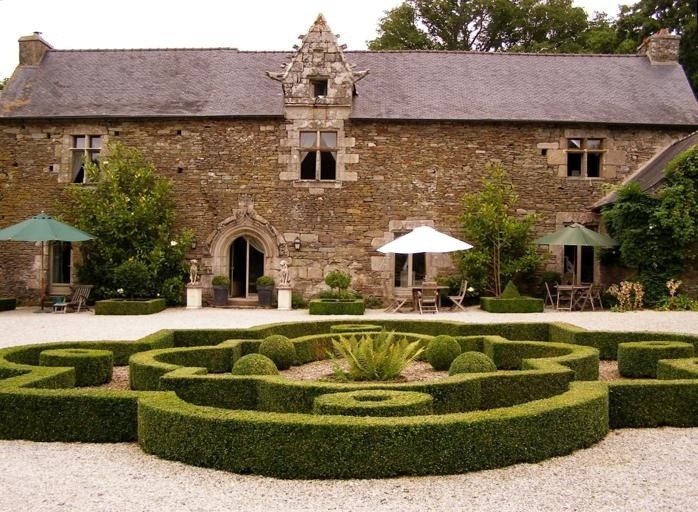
377;225;473;284
528;223;621;286
0;211;99;312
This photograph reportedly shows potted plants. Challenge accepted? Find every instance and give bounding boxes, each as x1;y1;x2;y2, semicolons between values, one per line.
212;275;229;305
257;276;275;305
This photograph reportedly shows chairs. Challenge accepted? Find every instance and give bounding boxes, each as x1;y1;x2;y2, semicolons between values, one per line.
38;283;93;313
380;276;469;314
544;280;605;312
